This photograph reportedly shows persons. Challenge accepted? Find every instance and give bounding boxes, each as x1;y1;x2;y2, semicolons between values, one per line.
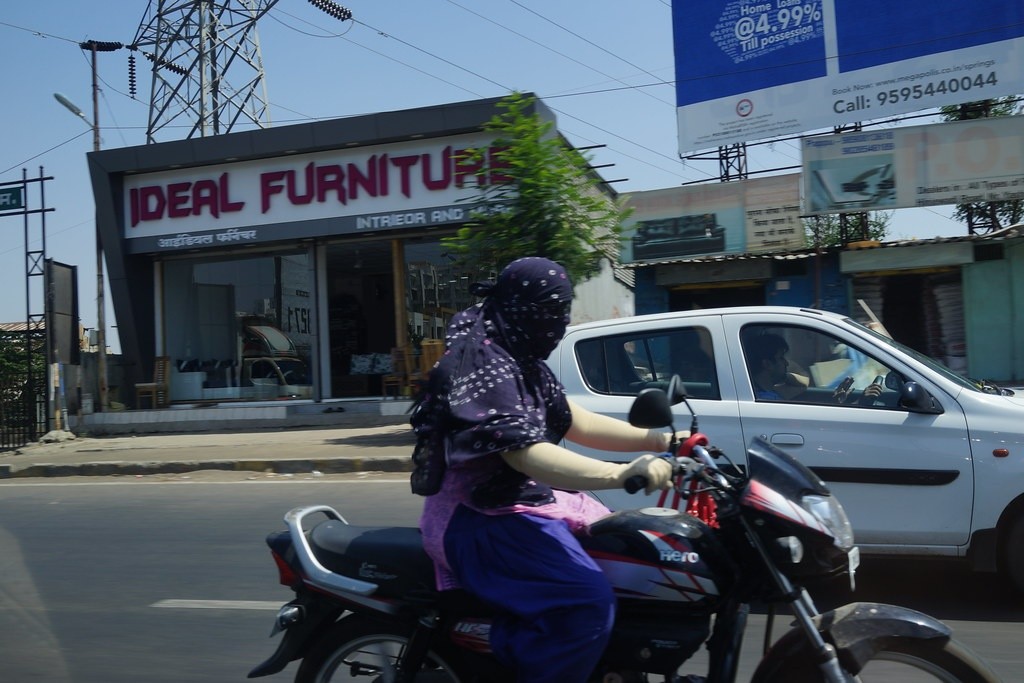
751;333;882;405
405;257;691;683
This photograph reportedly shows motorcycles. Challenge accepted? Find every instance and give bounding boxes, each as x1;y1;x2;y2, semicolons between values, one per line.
246;373;997;682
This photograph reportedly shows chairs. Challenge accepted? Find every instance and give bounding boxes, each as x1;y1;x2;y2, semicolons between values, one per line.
381;346;429;399
135;355;171;409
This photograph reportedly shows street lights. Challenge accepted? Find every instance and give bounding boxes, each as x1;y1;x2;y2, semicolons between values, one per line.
53;91;110;414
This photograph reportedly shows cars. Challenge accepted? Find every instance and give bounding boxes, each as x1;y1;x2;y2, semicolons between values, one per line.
546;305;1023;617
